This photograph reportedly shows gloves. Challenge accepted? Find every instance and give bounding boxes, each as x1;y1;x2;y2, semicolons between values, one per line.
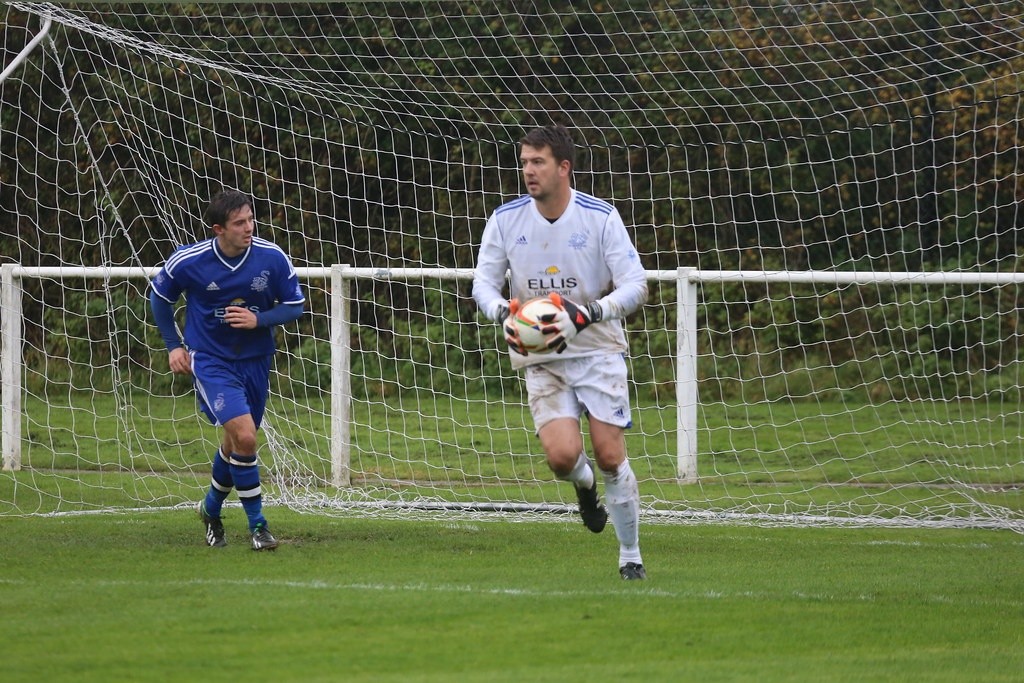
540;291;595;353
498;298;528;356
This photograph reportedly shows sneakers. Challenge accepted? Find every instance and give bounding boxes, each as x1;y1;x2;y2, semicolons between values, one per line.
249;520;278;550
198;497;227;547
574;458;608;533
619;561;647;580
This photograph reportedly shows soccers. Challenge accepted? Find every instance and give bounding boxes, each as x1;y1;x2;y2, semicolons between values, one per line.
512;297;566;354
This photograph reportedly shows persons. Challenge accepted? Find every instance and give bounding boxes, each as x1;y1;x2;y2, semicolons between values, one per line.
151;190;306;551
472;124;648;581
